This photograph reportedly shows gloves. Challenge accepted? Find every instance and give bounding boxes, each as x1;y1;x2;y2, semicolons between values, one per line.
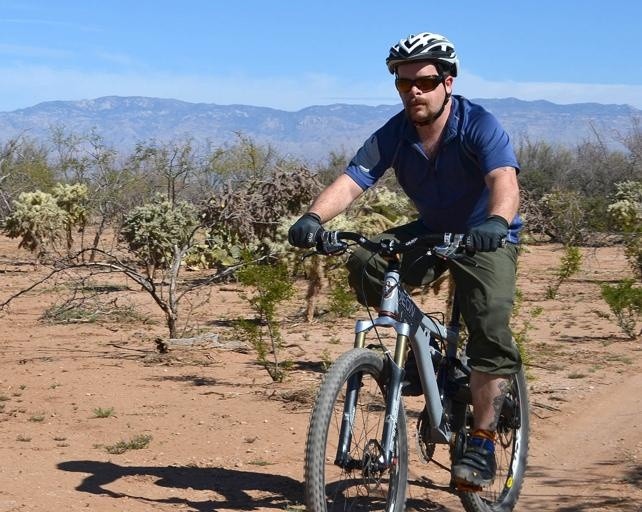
465;215;510;257
287;212;324;249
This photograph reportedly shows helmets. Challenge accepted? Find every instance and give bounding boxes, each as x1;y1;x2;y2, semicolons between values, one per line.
385;31;458;77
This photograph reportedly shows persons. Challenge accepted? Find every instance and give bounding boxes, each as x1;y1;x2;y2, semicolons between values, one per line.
287;31;527;495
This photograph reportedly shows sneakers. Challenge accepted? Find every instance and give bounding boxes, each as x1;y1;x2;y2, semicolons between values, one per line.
449;446;497;489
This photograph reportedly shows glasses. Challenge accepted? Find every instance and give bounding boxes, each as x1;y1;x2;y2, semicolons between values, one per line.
395;75;446;95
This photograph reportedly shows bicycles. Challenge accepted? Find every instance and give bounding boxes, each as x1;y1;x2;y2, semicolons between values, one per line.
288;224;528;510
